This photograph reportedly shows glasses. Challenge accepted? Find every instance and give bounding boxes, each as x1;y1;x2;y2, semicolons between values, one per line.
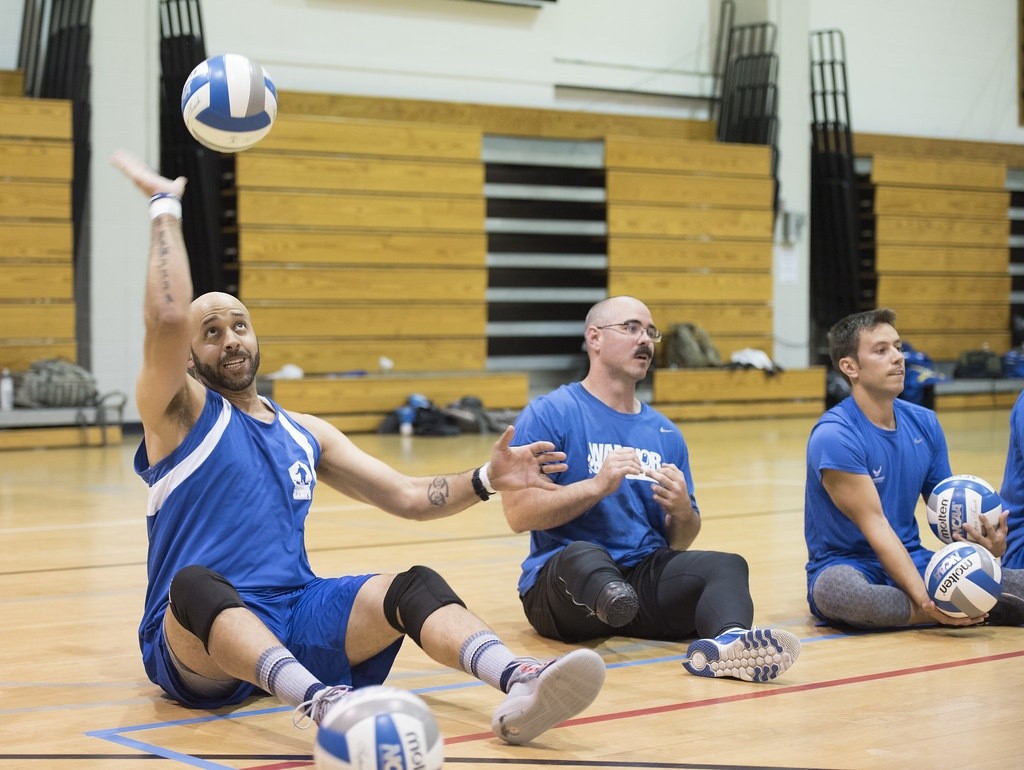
598;322;661;338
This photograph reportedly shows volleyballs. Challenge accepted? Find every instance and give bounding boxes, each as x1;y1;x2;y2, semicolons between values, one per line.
925;474;1004;544
179;52;281;152
922;542;1004;617
314;684;444;770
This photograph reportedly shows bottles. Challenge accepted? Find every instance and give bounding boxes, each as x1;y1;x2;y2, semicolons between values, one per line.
0;367;14;409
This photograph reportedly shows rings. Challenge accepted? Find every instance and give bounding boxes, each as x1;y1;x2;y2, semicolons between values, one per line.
539;466;545;475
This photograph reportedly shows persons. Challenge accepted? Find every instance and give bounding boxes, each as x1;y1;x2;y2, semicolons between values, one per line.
1000;388;1024;570
805;309;1024;635
502;296;802;683
109;149;605;747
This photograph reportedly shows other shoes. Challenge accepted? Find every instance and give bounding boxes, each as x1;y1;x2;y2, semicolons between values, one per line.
982;601;1024;626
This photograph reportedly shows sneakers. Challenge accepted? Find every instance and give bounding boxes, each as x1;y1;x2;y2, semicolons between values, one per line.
291;683;355;729
682;626;801;682
491;649;605;744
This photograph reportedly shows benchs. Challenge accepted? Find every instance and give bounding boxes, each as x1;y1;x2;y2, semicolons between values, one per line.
0;409;122;449
925;375;1023;409
636;366;826;422
258;370;530;431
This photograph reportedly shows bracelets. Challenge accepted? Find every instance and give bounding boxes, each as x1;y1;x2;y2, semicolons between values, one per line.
149;189;183;223
472;462;497;504
